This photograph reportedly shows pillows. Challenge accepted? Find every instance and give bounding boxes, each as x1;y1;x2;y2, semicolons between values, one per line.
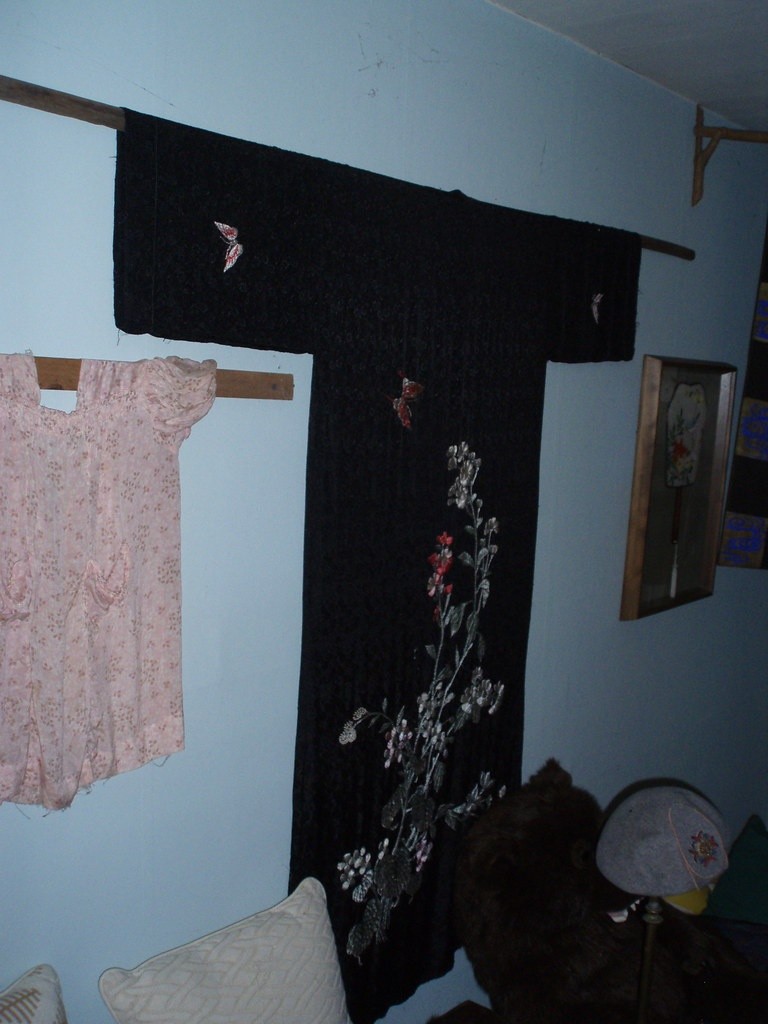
0;963;68;1024
98;877;354;1024
704;814;768;925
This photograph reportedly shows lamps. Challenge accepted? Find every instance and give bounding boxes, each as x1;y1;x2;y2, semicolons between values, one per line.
595;786;730;1024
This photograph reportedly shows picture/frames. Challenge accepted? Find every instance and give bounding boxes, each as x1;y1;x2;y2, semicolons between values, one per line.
620;355;737;621
